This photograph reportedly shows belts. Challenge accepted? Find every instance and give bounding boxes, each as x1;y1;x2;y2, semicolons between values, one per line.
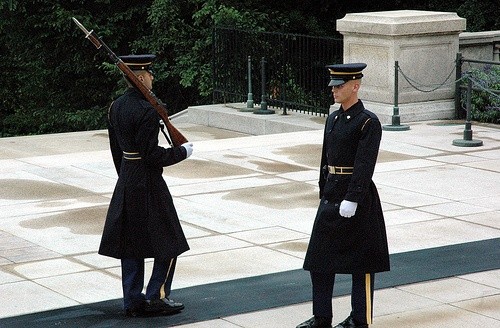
328;165;354;174
122;151;141;160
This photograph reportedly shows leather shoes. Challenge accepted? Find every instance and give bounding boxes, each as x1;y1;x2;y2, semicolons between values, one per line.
145;297;184;315
123;293;150;317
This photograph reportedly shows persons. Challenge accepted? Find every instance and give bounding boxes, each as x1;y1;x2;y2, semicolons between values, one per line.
98;54;193;316
296;62;391;328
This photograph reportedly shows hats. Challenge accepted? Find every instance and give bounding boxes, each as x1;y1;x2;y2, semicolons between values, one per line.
119;55;157;71
325;62;367;86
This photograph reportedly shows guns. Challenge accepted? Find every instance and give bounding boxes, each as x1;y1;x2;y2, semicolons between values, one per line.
72;16;188;147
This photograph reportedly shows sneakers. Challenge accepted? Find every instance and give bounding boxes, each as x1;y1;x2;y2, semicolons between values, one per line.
296;316;332;328
332;316;369;328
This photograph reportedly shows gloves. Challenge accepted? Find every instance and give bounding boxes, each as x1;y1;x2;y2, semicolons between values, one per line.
181;143;194;158
338;200;358;218
